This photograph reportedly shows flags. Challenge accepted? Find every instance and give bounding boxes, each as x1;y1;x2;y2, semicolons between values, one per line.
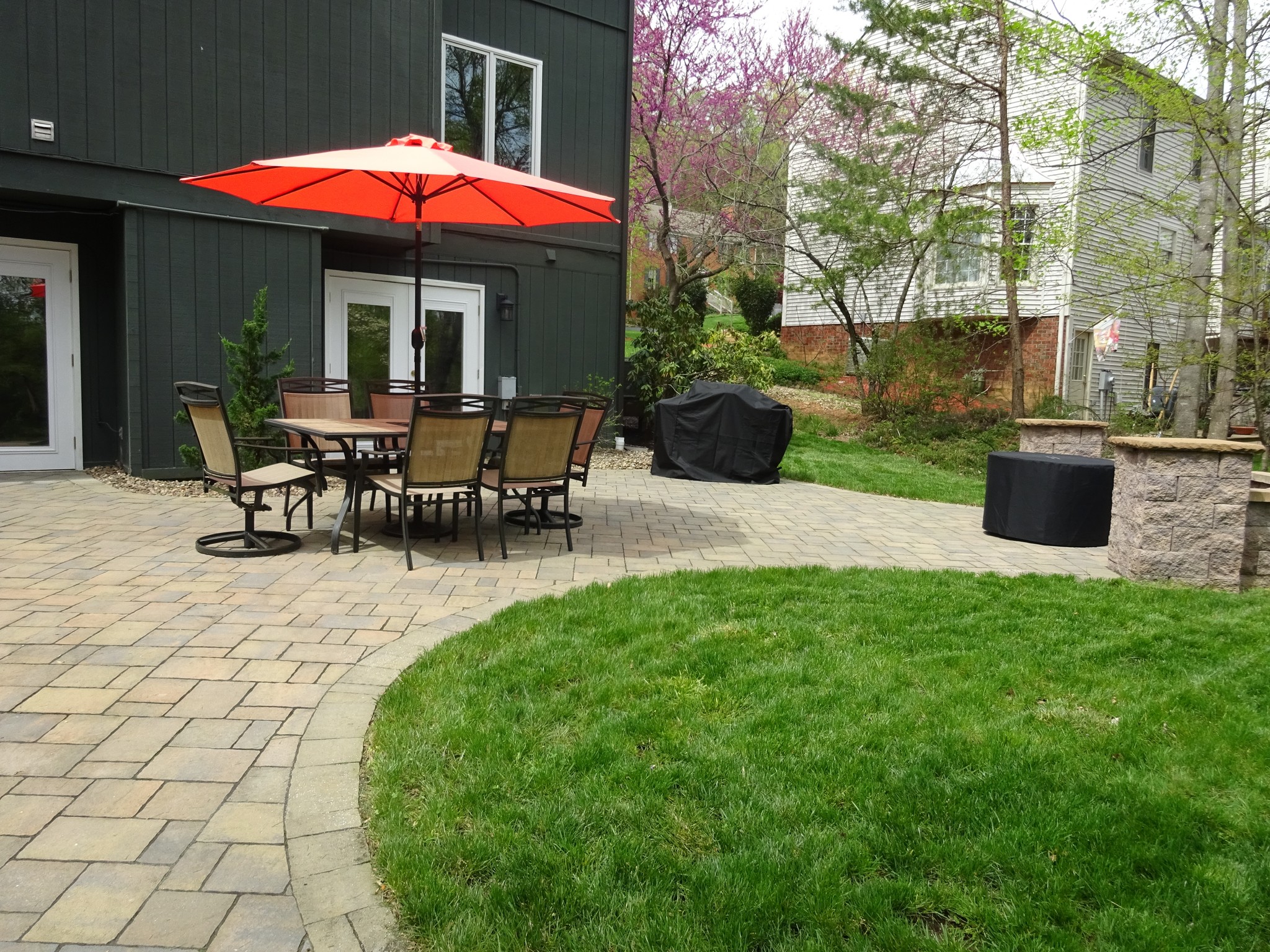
1093;308;1122;362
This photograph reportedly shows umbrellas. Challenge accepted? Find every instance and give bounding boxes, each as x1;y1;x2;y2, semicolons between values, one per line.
178;134;622;522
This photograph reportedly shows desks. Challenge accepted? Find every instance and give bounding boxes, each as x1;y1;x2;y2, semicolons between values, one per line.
980;450;1116;550
262;417;509;554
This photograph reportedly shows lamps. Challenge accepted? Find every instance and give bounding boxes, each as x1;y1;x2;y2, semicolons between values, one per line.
494;291;515;323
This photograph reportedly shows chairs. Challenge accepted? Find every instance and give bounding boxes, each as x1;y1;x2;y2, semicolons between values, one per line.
171;374;614;574
